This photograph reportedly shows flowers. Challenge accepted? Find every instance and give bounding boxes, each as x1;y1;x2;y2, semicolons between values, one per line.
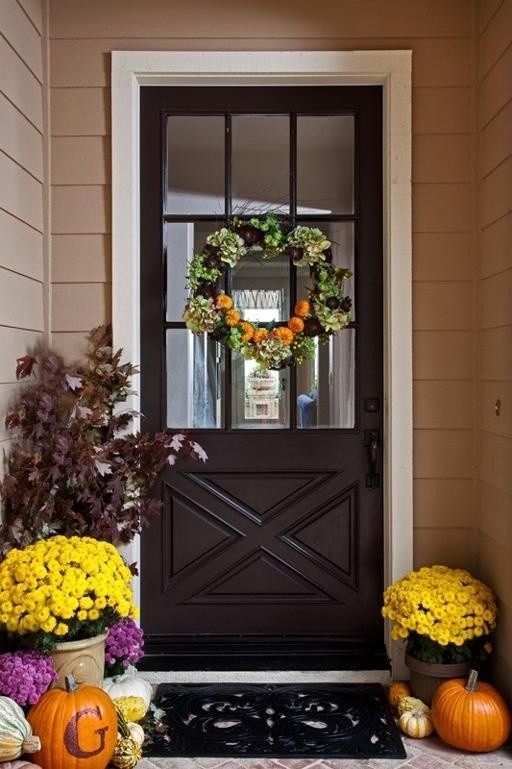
0;536;142;657
178;195;363;378
99;612;152;682
0;644;61;709
377;560;502;674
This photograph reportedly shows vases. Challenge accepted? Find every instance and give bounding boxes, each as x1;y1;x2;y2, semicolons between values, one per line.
39;625;108;696
401;649;475;709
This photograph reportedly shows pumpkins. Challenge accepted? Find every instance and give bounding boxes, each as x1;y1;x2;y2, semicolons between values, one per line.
0;674;151;769
216;294;310;346
388;669;512;753
